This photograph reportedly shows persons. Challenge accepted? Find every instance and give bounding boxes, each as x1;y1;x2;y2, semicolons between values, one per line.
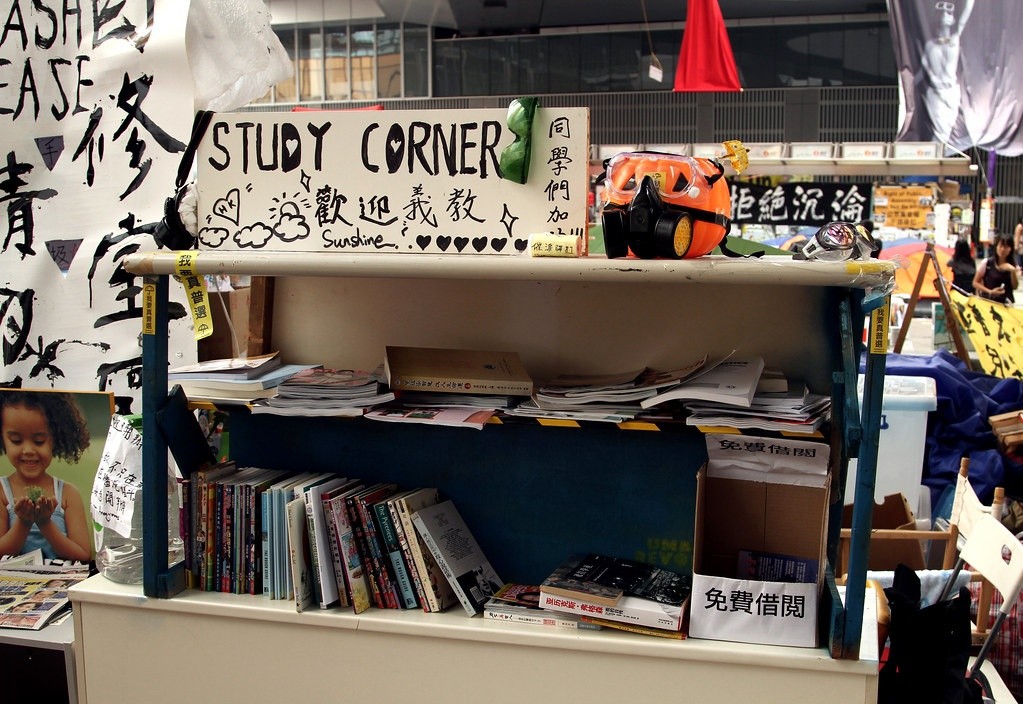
65;570;78;574
946;216;1023;307
472;566;501;599
0;391;93;560
45;580;68;588
517;592;541;604
310;369;354;385
23;590;58;601
7;601;43;613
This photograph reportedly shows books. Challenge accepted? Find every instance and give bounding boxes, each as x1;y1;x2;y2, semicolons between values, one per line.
0;547;89;631
173;344;834;640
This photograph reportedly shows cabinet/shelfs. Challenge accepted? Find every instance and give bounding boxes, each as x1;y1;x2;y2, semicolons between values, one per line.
121;253;896;661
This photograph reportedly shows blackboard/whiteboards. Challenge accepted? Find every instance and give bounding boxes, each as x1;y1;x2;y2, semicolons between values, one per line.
194;105;591;256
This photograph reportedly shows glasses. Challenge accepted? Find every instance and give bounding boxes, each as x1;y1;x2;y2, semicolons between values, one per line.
607;152;699;197
800;223;879;258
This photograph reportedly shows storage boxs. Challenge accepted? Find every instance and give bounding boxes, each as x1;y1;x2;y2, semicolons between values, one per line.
840;493;927;571
844;374;938;518
845;484;932;552
687;462;833;648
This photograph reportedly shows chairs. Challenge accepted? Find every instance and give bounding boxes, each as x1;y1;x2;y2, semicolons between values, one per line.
935;513;1023;679
842;457;1005;646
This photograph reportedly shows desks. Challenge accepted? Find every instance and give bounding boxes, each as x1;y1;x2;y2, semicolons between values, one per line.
65;577;879;704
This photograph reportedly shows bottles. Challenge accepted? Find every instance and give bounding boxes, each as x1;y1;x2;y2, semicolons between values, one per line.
91;415;184;585
